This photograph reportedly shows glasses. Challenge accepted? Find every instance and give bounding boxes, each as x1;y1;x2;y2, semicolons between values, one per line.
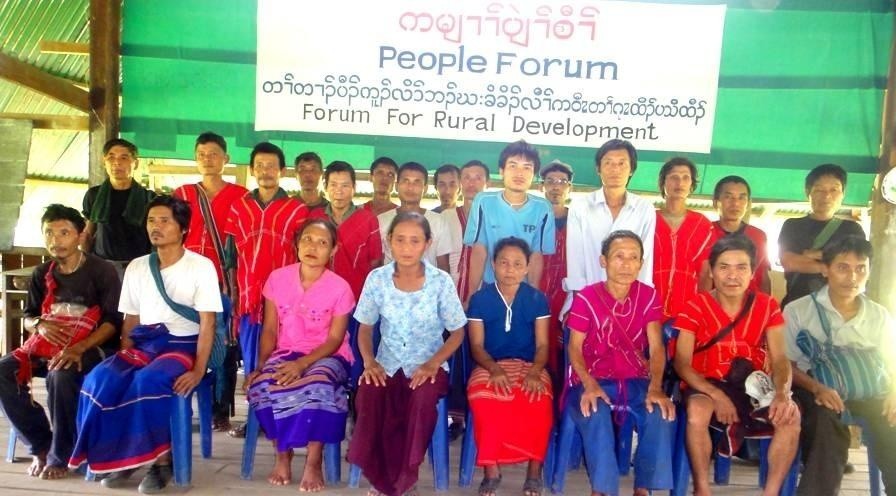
544;178;568;185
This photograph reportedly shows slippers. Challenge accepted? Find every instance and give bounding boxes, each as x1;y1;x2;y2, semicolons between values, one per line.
477;472;504;496
521;476;543;496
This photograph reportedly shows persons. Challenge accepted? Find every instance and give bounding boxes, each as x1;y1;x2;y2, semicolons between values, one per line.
780;164;866;312
0;202;122;480
782;234;896;496
68;194;225;494
173;132;800;495
80;140;159;270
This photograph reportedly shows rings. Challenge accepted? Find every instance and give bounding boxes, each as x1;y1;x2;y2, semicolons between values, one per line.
59;328;64;333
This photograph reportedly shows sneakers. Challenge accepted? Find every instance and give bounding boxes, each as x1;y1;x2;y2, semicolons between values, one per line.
447;423;465;444
100;465;140;489
227;422;247;439
138;456;174;495
211;415;231;433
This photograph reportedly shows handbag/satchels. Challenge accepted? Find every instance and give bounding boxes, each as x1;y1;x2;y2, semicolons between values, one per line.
22;302;102;358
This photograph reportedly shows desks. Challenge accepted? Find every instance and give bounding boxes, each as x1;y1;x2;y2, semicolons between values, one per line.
1;259;128;354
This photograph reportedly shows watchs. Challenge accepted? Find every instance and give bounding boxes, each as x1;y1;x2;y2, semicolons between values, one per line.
33;316;40;331
